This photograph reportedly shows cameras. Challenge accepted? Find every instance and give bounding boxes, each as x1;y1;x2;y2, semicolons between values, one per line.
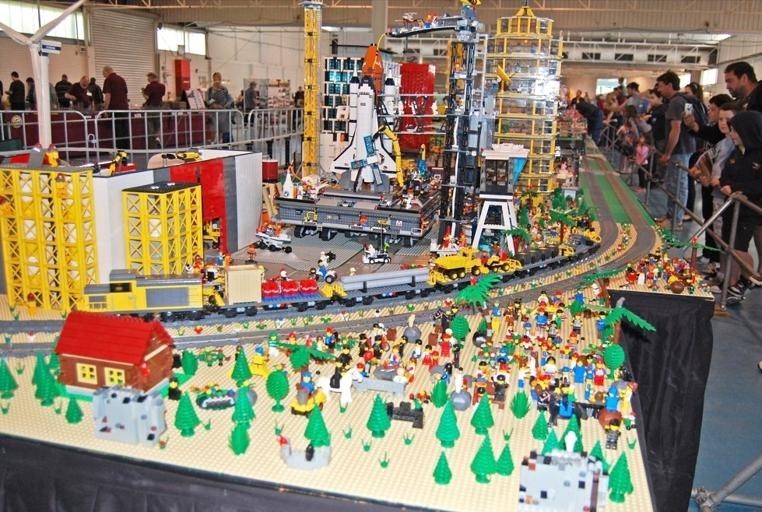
684;103;693;114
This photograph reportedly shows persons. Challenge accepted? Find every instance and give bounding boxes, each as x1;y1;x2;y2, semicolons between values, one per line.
572;78;668;192
655;71;708;230
0;72;36;110
48;68;129;148
239;82;258;127
141;73;165;146
207;73;233;150
294;87;303;107
691;61;762;304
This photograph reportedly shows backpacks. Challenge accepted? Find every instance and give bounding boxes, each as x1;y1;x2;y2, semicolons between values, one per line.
681;95;708;136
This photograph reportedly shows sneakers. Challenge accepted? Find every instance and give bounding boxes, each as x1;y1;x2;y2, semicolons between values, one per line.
710;281;723;293
727;276;755;296
636;187;646;194
699;257;711;263
658;220;683;231
702;260;718;275
683;214;693;222
654;215;666;221
749;273;762;287
621;167;632;174
